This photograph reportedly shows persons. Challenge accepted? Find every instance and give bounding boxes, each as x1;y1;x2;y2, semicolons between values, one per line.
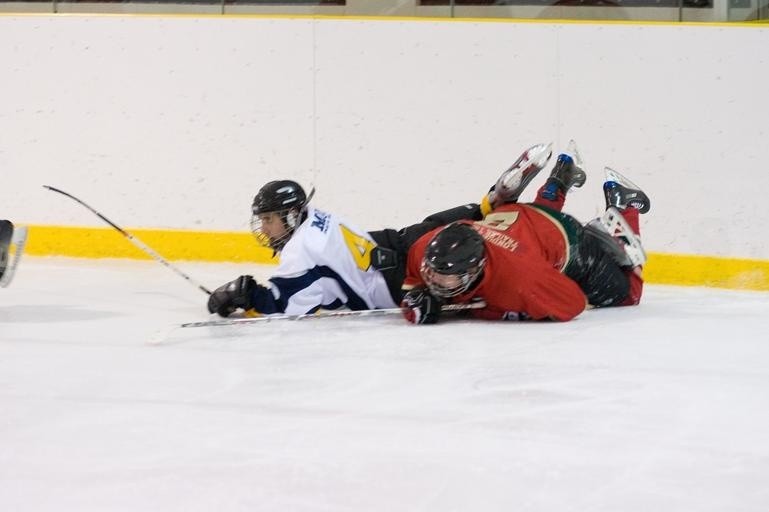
397;153;648;323
208;145;552;316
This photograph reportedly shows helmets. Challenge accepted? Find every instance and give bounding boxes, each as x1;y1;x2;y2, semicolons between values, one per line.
420;221;489;298
251;179;317;252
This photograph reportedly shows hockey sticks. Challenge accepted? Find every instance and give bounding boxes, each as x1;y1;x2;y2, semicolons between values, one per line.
44;185;213;296
146;301;486;345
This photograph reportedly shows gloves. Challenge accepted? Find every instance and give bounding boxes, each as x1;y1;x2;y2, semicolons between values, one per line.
401;285;442;325
209;274;258;318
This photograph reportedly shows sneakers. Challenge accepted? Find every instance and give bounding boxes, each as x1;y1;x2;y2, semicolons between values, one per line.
603;182;650;214
582;209;641;273
547;154;585;193
494;142;552;200
